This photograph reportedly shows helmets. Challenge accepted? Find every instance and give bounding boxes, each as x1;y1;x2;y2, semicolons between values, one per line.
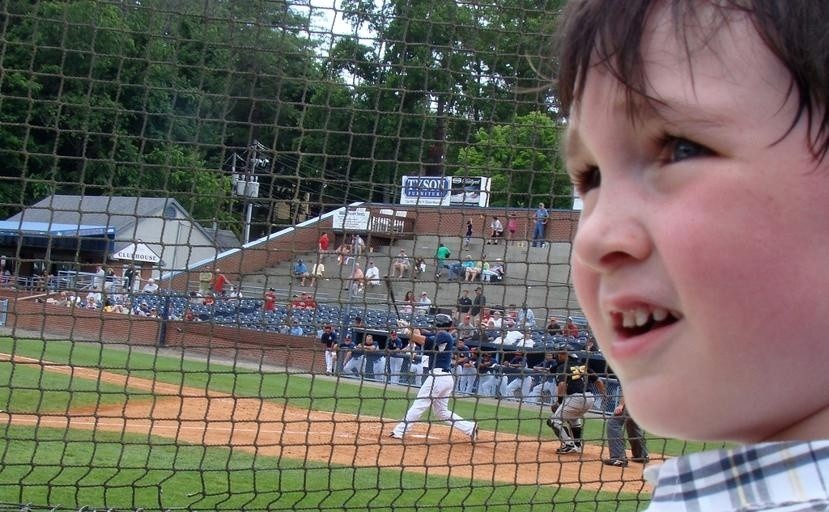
428;314;452;332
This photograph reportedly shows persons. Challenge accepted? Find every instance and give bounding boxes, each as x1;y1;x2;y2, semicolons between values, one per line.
600;387;652;469
563;316;580;339
487;214;505;246
463;217;474;252
551;3;829;512
320;325;338;376
451;337;571;408
547;317;565;337
357;334;381;379
532;203;550;249
401;339;424;388
388;314;481;448
552;401;583;455
508;214;521;247
339;333;368;380
39;232;537;348
384;331;405;387
545;351;607;455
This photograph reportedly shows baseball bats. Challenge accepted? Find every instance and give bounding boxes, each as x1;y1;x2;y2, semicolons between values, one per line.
383;275;400;321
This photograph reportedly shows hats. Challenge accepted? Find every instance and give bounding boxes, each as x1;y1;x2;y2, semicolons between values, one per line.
293;290;313;300
390;329;398;337
464;303;529;326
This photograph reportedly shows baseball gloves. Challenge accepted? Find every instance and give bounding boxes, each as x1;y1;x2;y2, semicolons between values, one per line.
550;401;559;414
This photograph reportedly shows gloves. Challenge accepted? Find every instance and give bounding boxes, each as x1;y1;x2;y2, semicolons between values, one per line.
395;319;409;336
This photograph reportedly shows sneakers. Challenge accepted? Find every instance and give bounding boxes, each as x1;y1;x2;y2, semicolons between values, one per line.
386;431;405;439
556;441;584;456
469;420;479;444
601;452;628;467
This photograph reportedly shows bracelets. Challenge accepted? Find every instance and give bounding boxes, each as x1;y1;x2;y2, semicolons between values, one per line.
557;395;565;406
